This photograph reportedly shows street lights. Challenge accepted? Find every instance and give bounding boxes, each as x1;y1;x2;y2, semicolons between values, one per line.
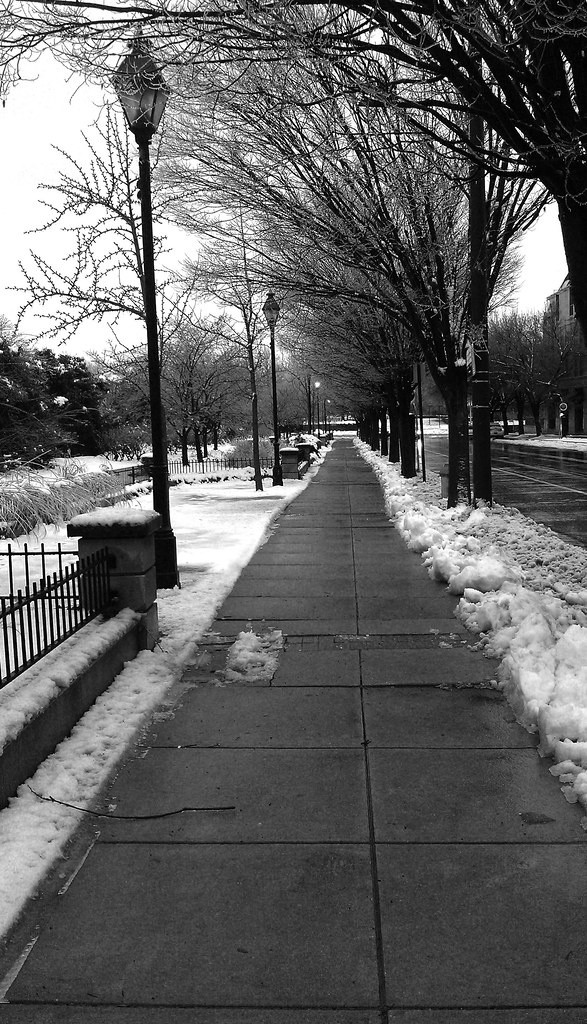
316;381;321;449
324;398;330;433
111;25;182;589
439;405;443;428
428;404;433;424
262;290;284;487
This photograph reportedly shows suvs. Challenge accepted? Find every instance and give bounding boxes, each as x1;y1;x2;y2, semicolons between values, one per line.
490;422;505;438
